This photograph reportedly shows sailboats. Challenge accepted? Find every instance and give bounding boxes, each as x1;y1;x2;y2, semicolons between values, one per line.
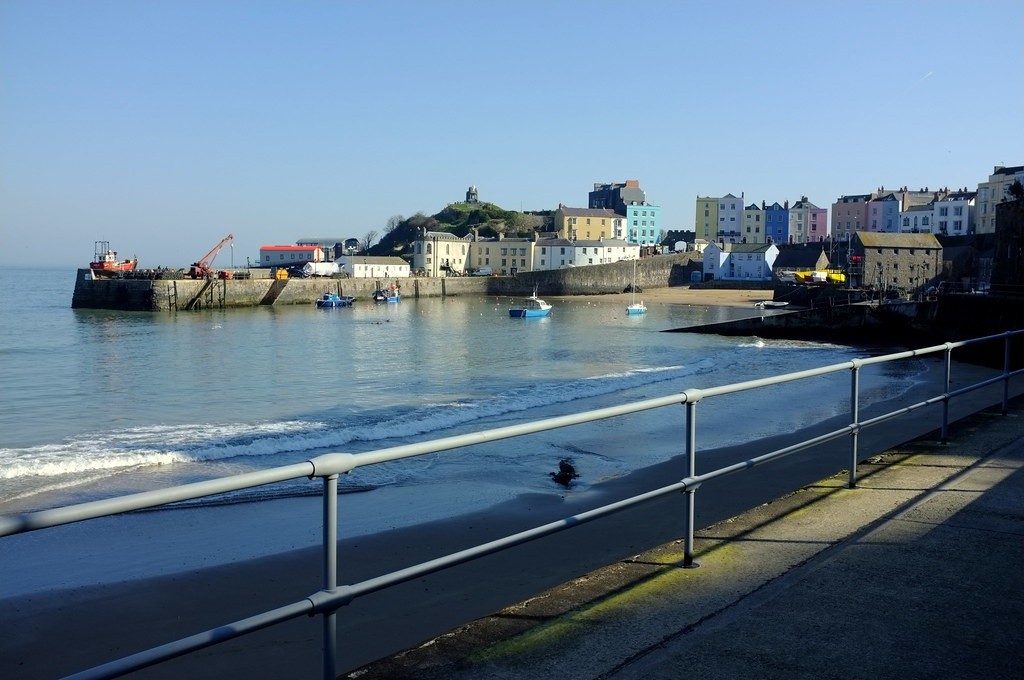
626;260;648;315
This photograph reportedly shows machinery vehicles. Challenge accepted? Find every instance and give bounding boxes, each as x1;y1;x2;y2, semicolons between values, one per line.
189;233;235;279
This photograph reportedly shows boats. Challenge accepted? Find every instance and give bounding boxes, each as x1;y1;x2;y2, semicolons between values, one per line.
316;291;358;308
508;287;556;319
375;285;403;304
89;240;139;272
755;300;790;310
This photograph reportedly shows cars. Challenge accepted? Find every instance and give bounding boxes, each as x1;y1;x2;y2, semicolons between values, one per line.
468;266;493;276
288;268;311;278
